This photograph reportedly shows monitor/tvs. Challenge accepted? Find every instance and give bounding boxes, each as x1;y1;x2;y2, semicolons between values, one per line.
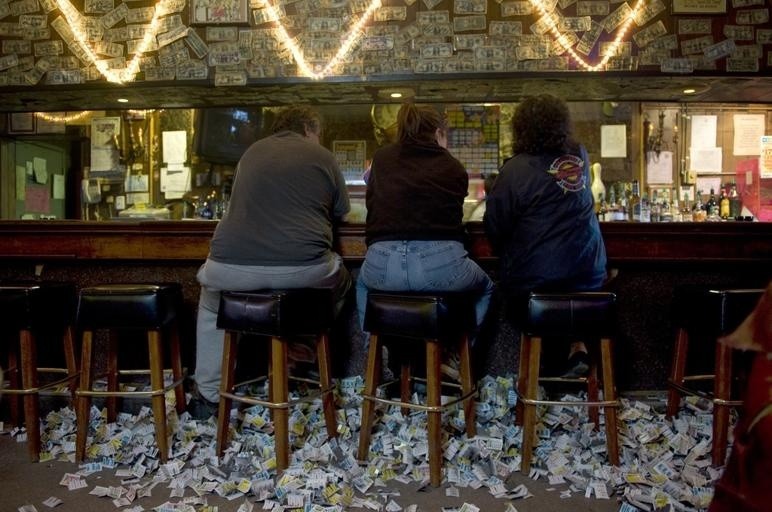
196;104;263;165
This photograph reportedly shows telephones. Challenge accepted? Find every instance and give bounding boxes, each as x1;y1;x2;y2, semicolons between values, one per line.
81;178;102;204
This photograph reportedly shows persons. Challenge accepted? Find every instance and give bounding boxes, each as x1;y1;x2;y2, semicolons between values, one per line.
194;109;353;417
360;101;495;382
484;97;609;377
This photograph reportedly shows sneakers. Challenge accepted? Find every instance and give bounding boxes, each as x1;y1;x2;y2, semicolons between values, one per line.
440;348;473;387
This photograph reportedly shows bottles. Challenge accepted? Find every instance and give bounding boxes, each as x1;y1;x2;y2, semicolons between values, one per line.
596;177;741;223
186;178;230;221
